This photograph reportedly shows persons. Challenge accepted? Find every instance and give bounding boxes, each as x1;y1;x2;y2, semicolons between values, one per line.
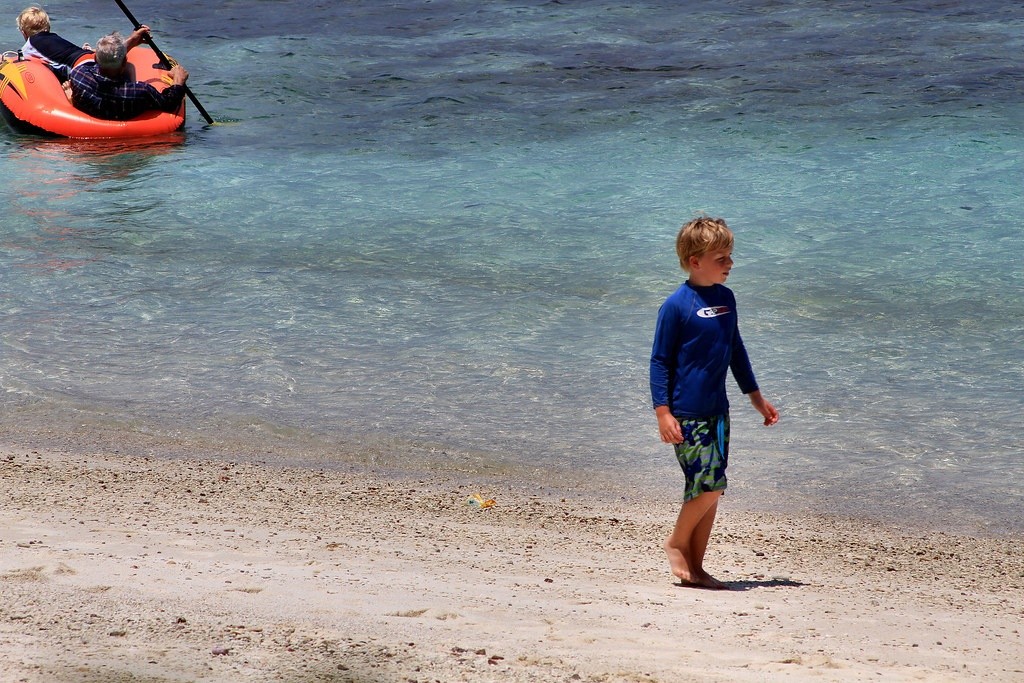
15;4;97;84
649;216;779;590
61;25;190;121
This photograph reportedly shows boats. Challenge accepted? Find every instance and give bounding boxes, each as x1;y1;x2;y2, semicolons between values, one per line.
0;46;186;139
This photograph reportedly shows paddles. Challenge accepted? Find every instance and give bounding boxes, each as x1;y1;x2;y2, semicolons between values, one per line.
115;0;215;125
